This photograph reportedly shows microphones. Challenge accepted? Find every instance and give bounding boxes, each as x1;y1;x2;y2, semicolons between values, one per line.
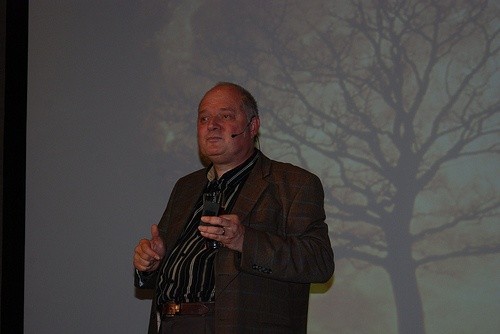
204;181;221;250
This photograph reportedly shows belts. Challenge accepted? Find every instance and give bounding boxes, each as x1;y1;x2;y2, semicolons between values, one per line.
160;301;214;315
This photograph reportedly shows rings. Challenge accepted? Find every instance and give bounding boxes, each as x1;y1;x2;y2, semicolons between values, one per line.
221;226;225;236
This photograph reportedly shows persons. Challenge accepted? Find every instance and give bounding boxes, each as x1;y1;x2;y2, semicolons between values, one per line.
132;82;336;333
